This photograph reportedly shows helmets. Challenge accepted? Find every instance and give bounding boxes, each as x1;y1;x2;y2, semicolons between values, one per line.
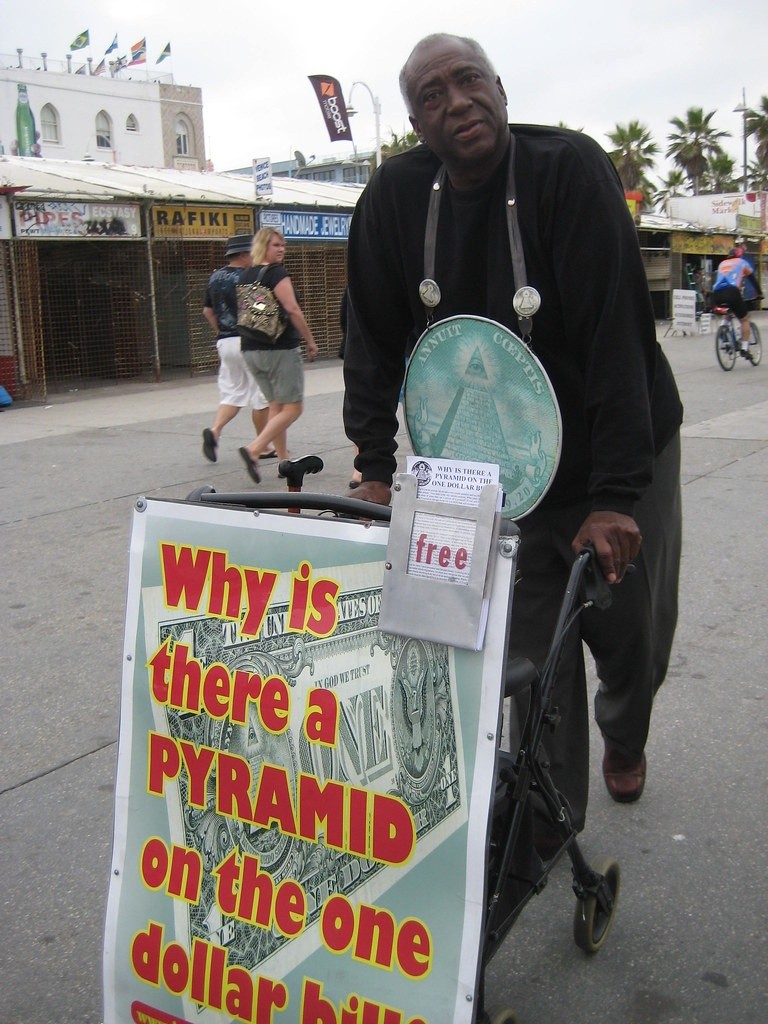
728;247;745;258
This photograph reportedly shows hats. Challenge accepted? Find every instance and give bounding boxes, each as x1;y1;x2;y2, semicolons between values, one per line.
223;234;254;256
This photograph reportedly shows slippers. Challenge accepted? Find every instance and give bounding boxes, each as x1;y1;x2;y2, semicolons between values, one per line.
202;427;221;461
259;448;277;458
239;446;264;484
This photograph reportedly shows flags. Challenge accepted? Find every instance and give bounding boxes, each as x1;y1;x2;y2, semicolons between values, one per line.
105;34;118;53
156;42;171;65
131;38;146;59
70;31;90;50
127;52;146;66
93;59;106;75
113;56;127;73
76;64;85;74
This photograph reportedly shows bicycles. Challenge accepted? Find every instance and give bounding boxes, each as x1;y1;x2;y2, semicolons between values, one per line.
711;296;763;371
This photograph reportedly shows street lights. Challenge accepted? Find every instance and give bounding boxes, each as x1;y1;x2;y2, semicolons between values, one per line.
345;81;382;169
732;87;749;192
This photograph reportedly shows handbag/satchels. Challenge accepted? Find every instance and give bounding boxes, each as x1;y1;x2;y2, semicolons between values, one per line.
235;265;281;336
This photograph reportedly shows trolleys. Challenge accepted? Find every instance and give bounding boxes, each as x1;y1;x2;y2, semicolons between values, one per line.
183;485;638;1024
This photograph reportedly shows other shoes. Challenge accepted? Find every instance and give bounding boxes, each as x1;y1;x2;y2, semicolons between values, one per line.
741;349;754;359
348;482;360;488
721;341;732;348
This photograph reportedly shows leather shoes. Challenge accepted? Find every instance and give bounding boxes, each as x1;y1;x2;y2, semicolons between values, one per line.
603;739;647;801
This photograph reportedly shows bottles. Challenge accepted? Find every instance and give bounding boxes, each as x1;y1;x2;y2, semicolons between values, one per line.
15;83;36;157
735;327;742;341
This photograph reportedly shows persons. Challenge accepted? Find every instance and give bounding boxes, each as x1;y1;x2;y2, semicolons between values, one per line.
340;32;683;861
224;227;317;482
711;247;764;360
202;233;289;461
